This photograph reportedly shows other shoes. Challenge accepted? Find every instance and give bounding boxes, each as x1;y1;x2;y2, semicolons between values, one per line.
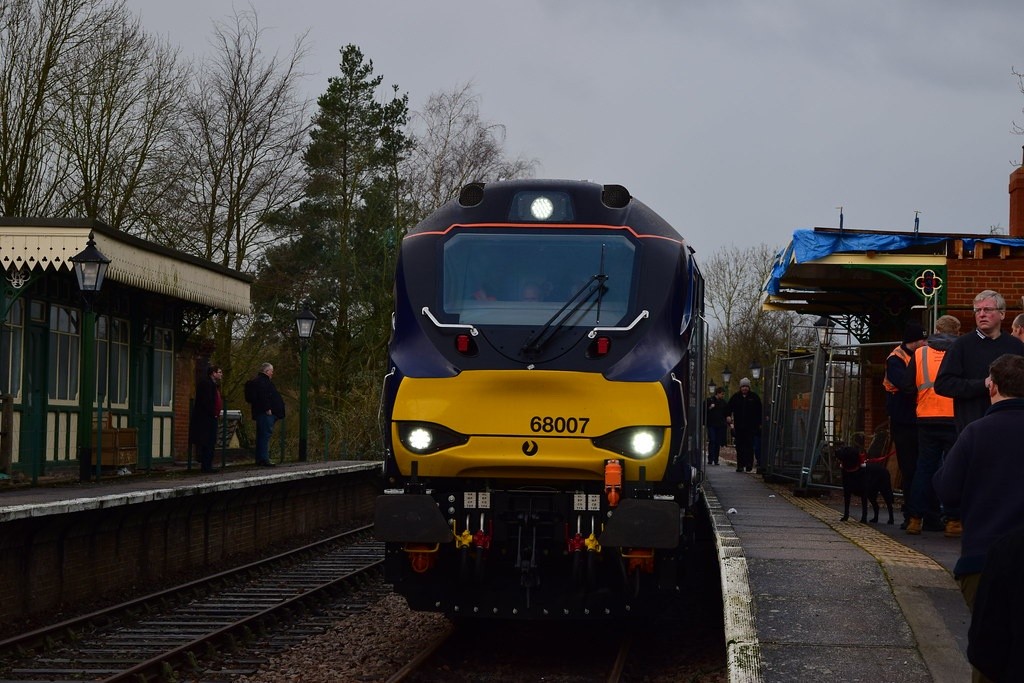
905;516;923;535
201;466;220;473
943;519;963;537
707;461;760;474
259;460;276;467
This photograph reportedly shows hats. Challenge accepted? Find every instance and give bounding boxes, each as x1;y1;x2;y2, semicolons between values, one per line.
903;319;928;343
740;377;750;389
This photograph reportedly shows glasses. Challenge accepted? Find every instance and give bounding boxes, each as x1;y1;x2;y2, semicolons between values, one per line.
974;306;1004;314
216;371;222;374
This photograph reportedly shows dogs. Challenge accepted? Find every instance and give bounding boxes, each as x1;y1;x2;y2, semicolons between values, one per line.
835;446;895;524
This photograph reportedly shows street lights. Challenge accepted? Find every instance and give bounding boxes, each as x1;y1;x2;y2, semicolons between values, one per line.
295;302;319;461
814;312;836;467
707;378;716;397
67;229;112;485
720;364;732;404
748;358;761;395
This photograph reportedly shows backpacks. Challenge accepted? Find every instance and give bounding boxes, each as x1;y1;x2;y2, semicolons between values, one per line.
244;379;252;404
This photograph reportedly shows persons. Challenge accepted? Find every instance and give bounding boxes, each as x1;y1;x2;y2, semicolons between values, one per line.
932;353;1024;683
934;289;1024;440
707;387;726;466
190;365;223;474
726;377;763;473
463;270;498;302
903;314;964;539
1010;313;1024;344
252;362;285;467
882;320;944;534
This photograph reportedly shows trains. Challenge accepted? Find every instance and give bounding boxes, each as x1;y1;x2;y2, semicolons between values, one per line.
378;179;710;639
